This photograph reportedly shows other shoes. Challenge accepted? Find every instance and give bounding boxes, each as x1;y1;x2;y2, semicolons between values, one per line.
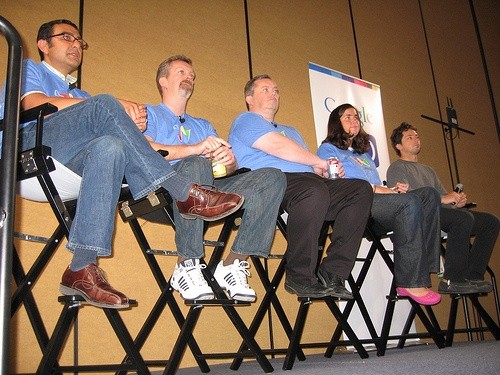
396;287;442;306
465;278;493;293
438;279;481;293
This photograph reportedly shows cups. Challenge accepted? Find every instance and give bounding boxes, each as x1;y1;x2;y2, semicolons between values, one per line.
212;159;227;178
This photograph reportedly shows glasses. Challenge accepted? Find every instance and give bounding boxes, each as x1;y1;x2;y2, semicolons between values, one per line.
43;32;88;49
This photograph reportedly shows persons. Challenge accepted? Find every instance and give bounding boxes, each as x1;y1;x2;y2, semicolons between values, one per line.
0;19;245;310
386;123;500;294
141;54;287;302
317;104;442;305
228;75;375;299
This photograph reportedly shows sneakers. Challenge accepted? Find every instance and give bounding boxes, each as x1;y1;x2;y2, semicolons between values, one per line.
317;257;354;300
284;273;334;298
170;258;214;300
178;184;245;222
59;263;129;308
213;258;256;303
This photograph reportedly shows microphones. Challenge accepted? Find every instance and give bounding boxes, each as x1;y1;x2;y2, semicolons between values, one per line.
452;183;463;205
68;83;76;90
179;116;185;123
273;123;277;128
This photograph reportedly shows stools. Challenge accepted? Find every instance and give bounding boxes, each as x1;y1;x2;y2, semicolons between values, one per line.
0;102;500;375
